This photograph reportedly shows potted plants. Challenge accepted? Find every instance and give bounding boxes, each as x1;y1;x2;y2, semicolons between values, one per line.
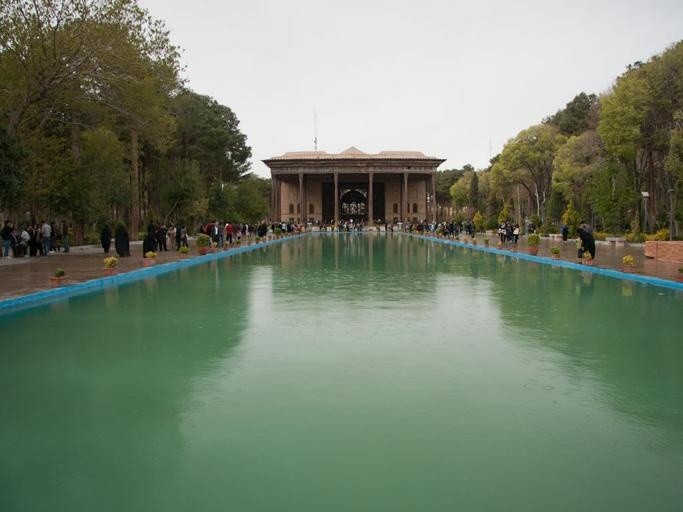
175;247;191;260
47;269;71;288
196;234;211;255
620;253;638;274
104;257;119;278
140;250;159;268
550;245;561;259
581;251;596;266
526;235;542;256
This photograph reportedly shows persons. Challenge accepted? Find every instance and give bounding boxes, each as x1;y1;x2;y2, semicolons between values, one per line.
0;218;69;257
198;220;315;250
560;219;595;263
317;216;397;232
148;219;189;251
497;220;521;249
404;219;476;244
